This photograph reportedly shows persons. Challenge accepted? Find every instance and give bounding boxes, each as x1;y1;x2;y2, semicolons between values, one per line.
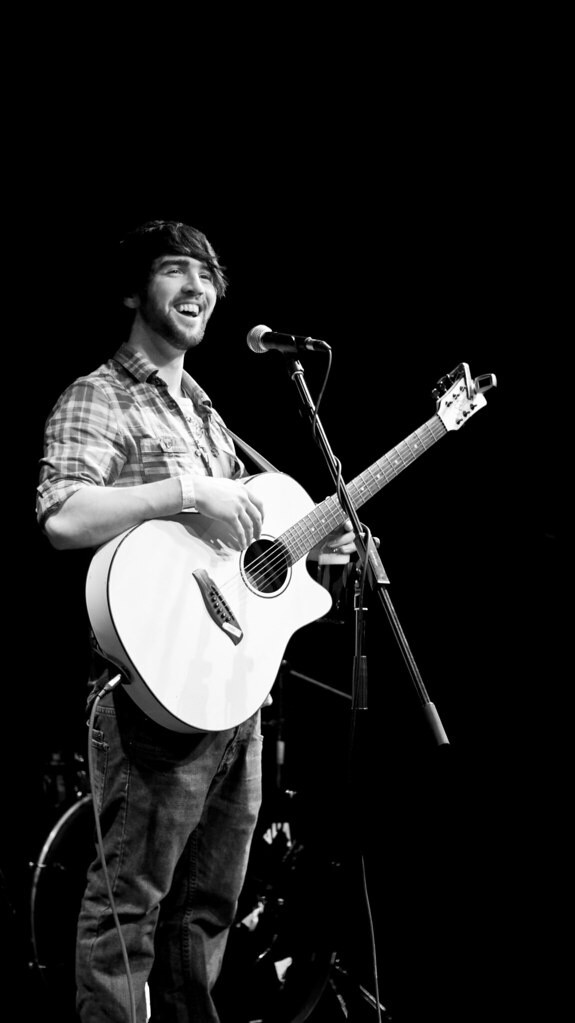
35;220;381;1023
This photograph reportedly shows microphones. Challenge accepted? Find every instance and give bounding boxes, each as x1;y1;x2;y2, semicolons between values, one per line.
247;325;332;353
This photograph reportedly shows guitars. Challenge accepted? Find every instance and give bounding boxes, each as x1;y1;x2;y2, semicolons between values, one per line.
85;363;490;740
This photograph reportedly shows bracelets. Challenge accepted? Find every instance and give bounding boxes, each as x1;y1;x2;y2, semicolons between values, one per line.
181;482;196;509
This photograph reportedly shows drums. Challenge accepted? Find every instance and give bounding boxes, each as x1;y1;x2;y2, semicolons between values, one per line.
30;790;339;1023
